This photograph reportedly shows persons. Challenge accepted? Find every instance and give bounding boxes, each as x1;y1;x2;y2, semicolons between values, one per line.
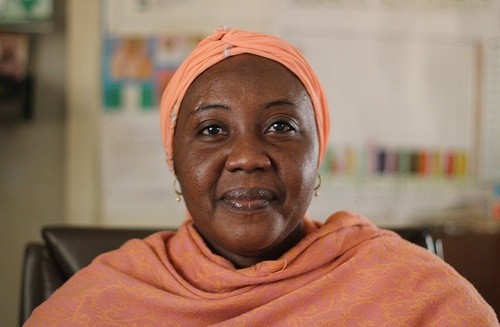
22;27;499;327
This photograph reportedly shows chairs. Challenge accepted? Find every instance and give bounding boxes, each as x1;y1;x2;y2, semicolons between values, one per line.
18;224;448;326
